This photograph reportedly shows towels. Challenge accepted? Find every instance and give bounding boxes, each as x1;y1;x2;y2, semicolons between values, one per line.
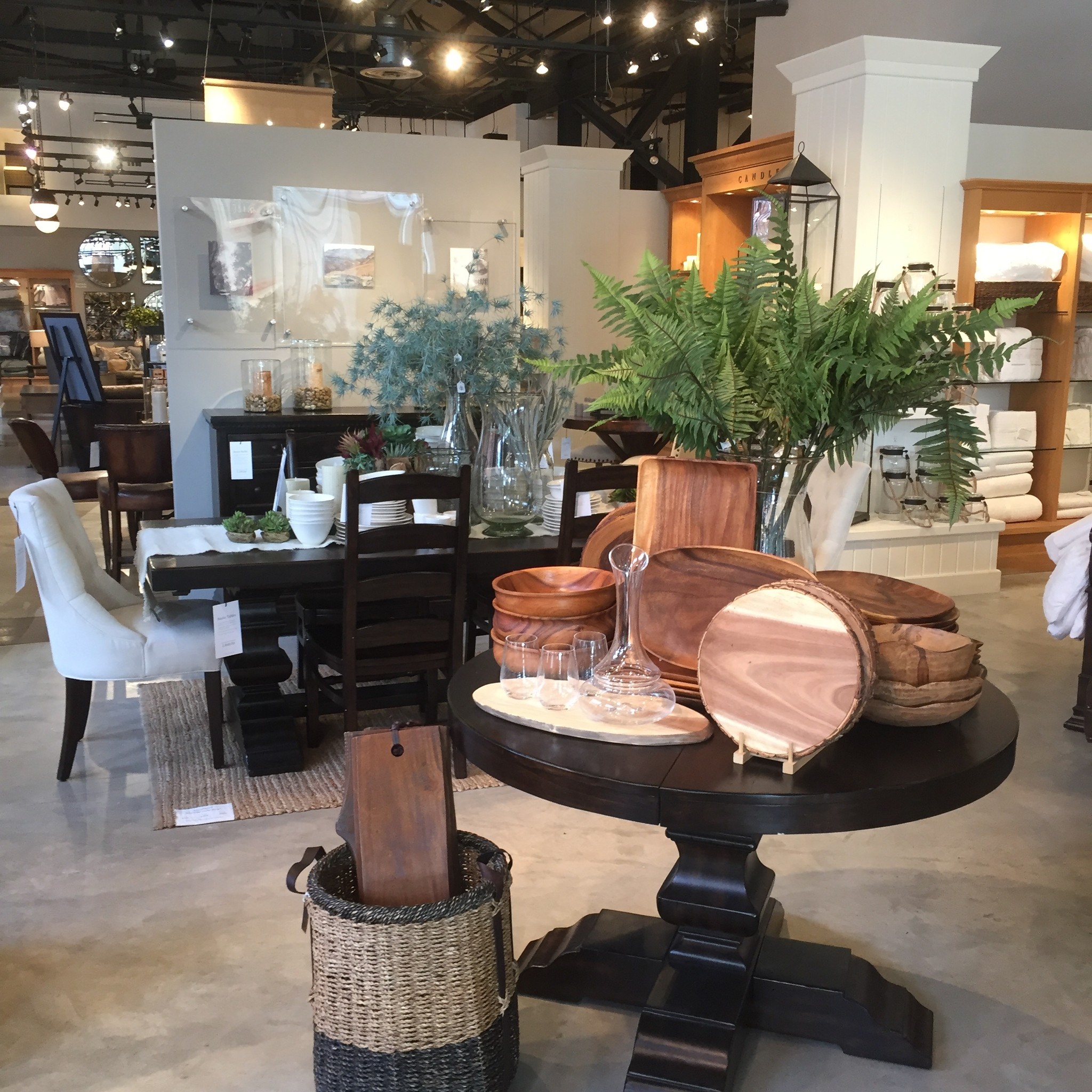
962;449;1043;522
1056;490;1092;519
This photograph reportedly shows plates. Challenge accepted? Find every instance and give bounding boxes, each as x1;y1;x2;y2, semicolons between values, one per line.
542;490;615;532
334;469;413;541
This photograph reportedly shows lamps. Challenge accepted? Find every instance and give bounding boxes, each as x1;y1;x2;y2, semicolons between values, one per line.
16;84;156;234
111;0;716;77
342;116;358;132
29;330;49;365
648;149;659;165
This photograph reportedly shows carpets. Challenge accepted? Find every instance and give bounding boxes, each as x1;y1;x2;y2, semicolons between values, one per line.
137;681;511;830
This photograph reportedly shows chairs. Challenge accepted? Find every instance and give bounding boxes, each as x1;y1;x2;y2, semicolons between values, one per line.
8;417;138;554
8;476;227;780
94;422;173;585
286;428;638;776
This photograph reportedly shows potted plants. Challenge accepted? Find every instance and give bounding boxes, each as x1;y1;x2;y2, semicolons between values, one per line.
327;230;1065;590
123;308;163;346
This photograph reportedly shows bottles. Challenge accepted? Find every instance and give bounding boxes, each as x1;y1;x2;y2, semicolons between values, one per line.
874;445;989;526
579;542;676;727
871;262;975;360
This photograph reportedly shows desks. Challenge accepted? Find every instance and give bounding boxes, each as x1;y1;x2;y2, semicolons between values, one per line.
138;517;587;781
27;364;47;385
447;650;1020;1091
563;416;674;460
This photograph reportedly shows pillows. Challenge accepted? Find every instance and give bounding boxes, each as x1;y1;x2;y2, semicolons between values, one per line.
89;343;143;369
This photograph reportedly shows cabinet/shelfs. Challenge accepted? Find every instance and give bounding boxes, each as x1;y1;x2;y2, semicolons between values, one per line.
201;407;480;517
661;130;795;294
948;178;1092;574
0;267;76;395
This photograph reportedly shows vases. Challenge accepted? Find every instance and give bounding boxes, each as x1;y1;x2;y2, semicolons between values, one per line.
470;392;547;537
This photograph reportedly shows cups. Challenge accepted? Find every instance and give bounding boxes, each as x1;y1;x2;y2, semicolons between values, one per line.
143;377;170;423
537;643;579;711
471;394;544;537
481;466;529;510
285;478;315;519
290;338;333;410
499;633;538;699
242;359;281;413
412;497;457;527
567;632;609;693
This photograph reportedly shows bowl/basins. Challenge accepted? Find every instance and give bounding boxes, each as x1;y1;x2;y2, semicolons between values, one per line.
288;494;335;546
860;623;989;726
420;448;471;477
316;455;345;494
412;426;446;448
547;479;565;500
490;566;626;680
580;455;959;759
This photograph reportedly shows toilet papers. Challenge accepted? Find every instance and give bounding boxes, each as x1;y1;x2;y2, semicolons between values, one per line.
151;391;168;424
